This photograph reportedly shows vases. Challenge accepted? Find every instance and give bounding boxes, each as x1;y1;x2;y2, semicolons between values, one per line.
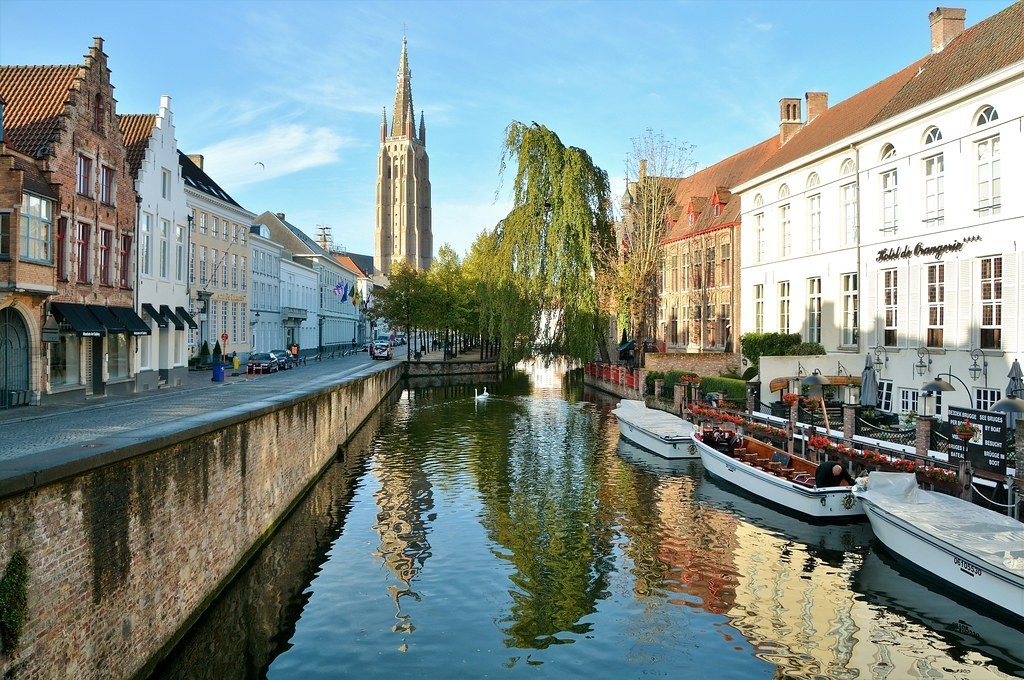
826;451;962;492
957;432;974;439
681;380;689;385
690;382;700;388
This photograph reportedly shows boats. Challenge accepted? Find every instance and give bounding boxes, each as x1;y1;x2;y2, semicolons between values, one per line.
849;545;1024;678
611;399;701;460
693;470;875;560
689;423;867;517
615;433;701;475
852;470;1024;618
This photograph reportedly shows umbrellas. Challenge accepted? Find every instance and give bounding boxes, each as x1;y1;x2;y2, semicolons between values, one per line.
861;352;879;410
1006;359;1024;420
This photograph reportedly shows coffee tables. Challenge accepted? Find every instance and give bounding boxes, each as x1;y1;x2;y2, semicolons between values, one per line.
791;470;807;482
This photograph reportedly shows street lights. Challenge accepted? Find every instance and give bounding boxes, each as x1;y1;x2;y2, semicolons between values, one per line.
921;373;974;409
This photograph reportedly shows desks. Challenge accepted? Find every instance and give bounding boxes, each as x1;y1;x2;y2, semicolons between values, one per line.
890;425;916;429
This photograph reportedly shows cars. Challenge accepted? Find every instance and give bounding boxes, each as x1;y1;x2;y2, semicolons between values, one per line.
247;352;278;373
270;349;294;370
362;342;371;351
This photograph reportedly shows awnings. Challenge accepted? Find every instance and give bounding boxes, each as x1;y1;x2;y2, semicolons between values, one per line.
142;303;198;331
50;302;151;337
616;340;635;350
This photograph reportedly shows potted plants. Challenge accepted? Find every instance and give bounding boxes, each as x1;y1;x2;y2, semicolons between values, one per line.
801;384;811;398
746;428;788;443
822;385;837;398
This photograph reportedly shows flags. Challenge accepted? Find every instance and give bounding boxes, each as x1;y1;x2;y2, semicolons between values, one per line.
333;279;369;311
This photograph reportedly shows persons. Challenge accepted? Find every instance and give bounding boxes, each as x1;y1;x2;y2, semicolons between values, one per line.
433;339;437;351
815;461;856;488
352;342;356;350
369;339;373;343
437;341;441;351
292;344;299;358
401;338;403;347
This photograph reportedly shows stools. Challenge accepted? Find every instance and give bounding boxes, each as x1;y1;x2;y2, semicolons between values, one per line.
781;468;795;479
767;461;782;476
756;458;770;472
745;452;758;466
734;448;746;461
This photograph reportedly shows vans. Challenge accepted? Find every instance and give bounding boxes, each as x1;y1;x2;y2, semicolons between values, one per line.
396;331;407;345
368;342;393;360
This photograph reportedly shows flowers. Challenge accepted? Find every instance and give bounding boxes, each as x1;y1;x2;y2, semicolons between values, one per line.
693;375;703;382
687;401;786;436
807;436;958;483
954;419;982;442
679;374;692;382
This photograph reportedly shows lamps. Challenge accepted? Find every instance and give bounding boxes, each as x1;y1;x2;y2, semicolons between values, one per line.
968;349;987;381
915;347;932;377
873;346;889;373
